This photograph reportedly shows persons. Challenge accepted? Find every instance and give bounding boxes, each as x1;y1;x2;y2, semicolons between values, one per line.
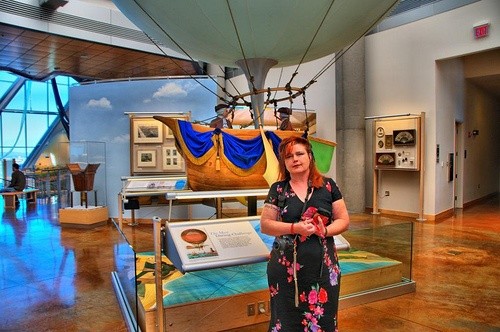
260;135;350;332
0;163;26;205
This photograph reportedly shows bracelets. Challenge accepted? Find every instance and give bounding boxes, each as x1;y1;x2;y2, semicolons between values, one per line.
324;227;327;237
291;222;295;235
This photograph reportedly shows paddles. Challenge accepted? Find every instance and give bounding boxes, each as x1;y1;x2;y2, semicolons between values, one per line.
257;106;282;188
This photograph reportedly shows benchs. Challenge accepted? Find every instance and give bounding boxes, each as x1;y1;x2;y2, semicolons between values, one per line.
2;189;39;208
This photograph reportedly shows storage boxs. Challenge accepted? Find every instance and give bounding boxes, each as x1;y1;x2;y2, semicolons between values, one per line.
59;207;109;230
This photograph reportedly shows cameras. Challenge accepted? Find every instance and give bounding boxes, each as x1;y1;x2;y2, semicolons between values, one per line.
273;235;294;251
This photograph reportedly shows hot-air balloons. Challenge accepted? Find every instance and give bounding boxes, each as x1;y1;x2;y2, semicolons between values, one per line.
113;0;400;191
